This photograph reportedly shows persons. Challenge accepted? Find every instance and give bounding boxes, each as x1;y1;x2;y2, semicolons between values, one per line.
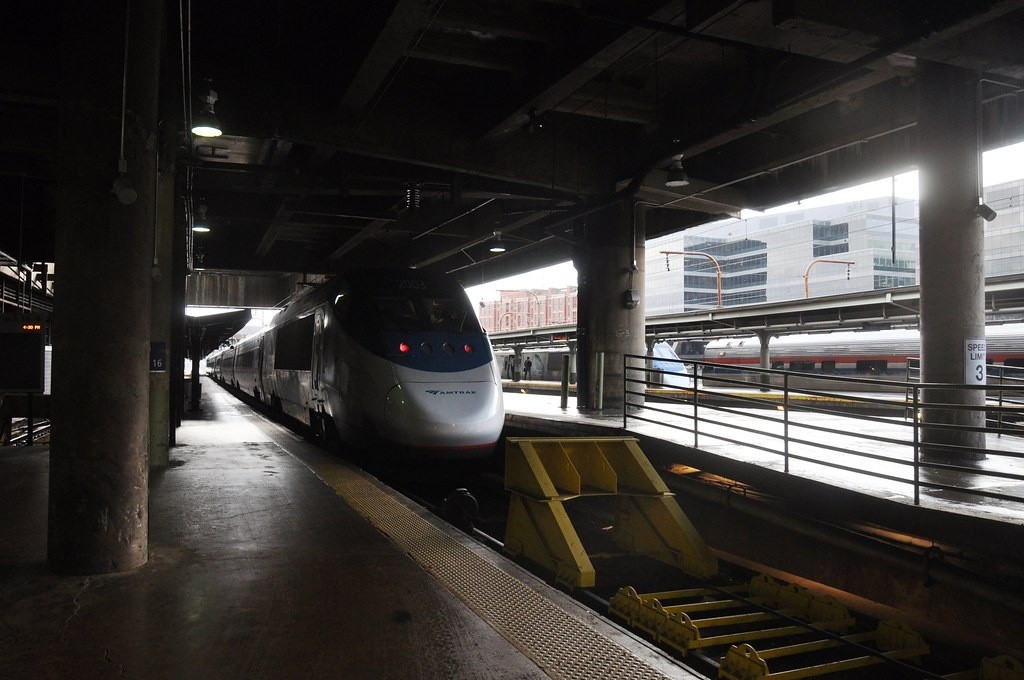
511;356;515;380
524;357;532;380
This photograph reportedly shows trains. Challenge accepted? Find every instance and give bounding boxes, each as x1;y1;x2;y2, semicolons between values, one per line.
697;323;1023;414
494;340;694;406
204;264;508;508
